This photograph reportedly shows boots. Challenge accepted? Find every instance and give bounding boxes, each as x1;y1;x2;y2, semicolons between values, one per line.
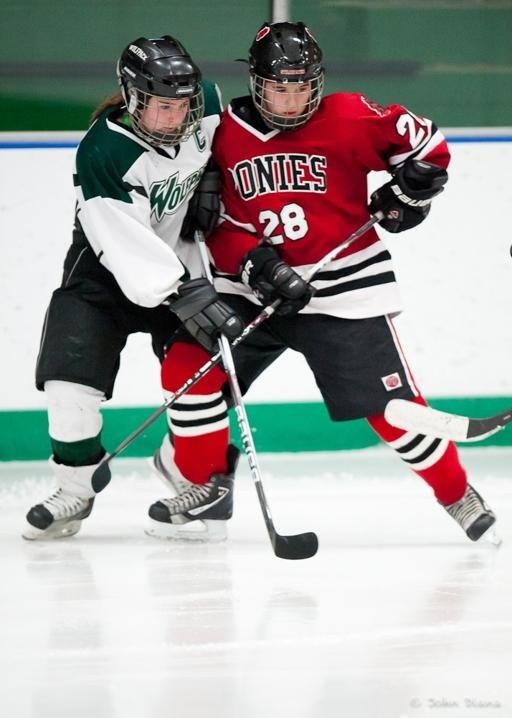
149;444;239;524
442;482;492;541
153;433;194;497
26;453;109;530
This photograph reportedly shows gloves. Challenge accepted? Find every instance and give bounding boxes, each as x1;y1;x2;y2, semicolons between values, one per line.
169;276;245;355
180;170;222;243
368;160;448;233
241;246;311;319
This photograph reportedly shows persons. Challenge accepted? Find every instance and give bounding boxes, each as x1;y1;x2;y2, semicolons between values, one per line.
148;19;494;542
27;39;225;532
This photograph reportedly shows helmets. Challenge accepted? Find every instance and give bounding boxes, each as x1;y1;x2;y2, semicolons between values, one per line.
116;34;205;148
247;20;325;132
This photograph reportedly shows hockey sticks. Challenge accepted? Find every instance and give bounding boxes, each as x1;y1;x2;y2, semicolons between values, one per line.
91;209;385;493
196;225;318;558
383;398;511;444
